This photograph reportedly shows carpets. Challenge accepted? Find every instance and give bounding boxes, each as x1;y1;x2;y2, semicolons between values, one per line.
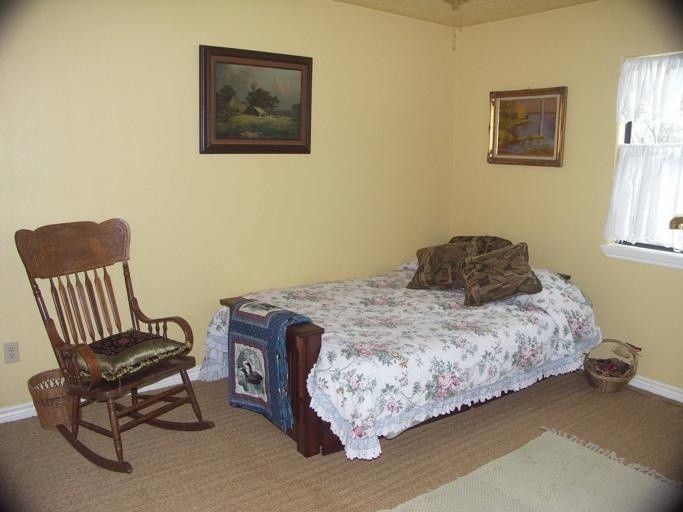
377;426;683;512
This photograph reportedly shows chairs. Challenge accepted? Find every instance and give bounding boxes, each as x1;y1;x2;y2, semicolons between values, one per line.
15;218;216;474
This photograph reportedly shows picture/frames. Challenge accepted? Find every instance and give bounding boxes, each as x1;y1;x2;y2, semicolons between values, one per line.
199;45;314;155
486;86;568;167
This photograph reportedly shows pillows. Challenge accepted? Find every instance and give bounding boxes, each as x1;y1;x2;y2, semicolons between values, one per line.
78;328;191;383
405;235;543;306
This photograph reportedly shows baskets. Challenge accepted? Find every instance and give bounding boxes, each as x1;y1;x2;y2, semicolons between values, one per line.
583;338;638;393
28;368;81;431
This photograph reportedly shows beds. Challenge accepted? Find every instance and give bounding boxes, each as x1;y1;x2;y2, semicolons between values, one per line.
222;274;604;458
